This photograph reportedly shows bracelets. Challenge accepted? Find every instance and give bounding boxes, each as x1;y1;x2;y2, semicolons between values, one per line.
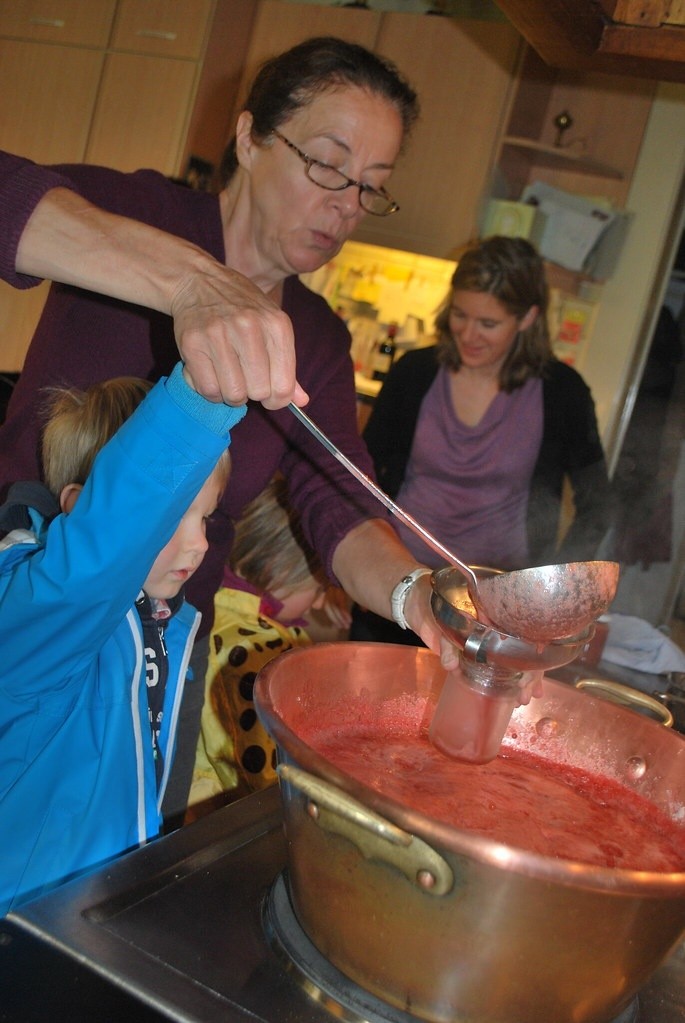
391;566;434;631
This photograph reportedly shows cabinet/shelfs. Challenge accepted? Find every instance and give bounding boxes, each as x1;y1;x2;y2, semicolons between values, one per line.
0;0;257;371
475;40;660;295
224;5;527;262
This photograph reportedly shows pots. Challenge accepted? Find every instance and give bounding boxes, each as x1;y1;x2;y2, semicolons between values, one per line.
253;637;685;1022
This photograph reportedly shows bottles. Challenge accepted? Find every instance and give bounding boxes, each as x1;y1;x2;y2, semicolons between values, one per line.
577;615;612;669
425;654;524;766
369;325;398;381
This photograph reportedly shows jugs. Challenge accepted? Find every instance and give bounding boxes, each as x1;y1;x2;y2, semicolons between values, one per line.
342;300;379;372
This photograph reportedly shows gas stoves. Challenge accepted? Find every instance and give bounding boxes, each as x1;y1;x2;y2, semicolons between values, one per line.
4;782;685;1021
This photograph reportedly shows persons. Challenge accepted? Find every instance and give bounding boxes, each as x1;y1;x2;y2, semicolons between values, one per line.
0;36;545;837
0;359;251;917
326;234;618;650
182;471;337;825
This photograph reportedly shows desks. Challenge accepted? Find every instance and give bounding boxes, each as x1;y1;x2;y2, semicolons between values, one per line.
4;620;685;1023
